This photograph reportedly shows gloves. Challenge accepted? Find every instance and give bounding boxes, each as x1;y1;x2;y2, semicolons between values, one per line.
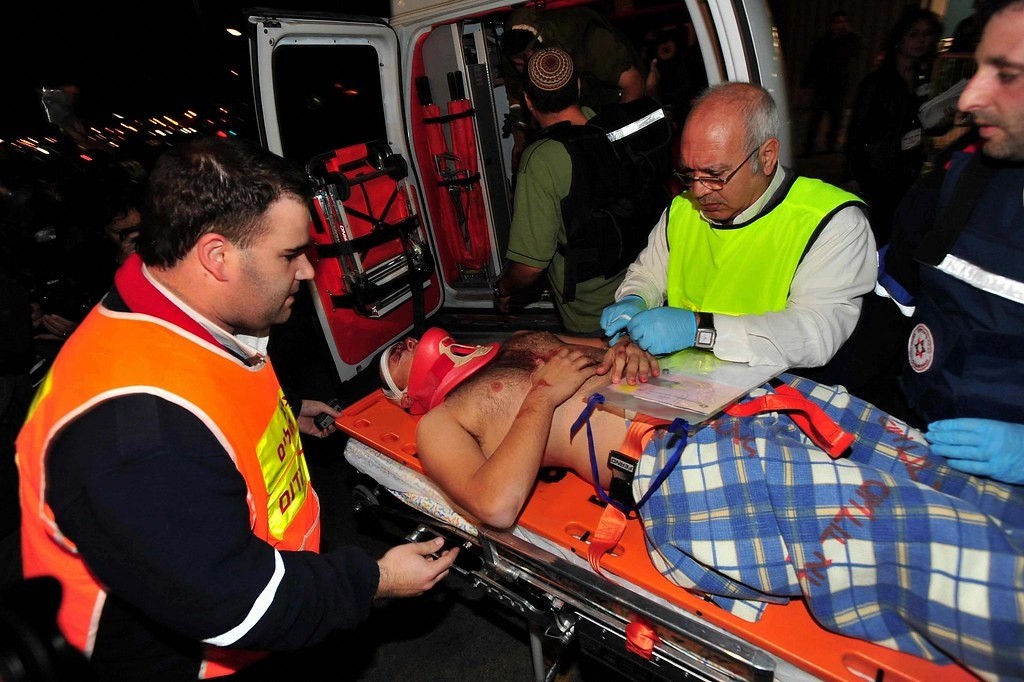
600;293;648;346
626;308;697;355
924;417;1024;486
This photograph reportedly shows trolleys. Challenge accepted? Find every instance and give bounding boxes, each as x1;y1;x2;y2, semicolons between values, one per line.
333;385;983;682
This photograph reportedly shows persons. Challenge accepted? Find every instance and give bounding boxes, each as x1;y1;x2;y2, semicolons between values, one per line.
0;0;1024;682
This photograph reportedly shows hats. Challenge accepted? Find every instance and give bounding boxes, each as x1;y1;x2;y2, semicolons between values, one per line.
528;46;574;92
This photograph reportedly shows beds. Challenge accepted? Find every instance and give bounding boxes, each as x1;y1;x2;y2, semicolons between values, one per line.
335;385;986;682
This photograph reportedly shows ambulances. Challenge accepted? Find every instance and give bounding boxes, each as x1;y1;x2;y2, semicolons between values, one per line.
244;1;797;383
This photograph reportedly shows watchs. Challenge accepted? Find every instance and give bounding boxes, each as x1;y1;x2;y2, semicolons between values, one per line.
695;312;716;351
493;284;509;298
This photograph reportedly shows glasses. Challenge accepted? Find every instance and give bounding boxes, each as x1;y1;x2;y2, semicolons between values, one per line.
672;143;763;190
